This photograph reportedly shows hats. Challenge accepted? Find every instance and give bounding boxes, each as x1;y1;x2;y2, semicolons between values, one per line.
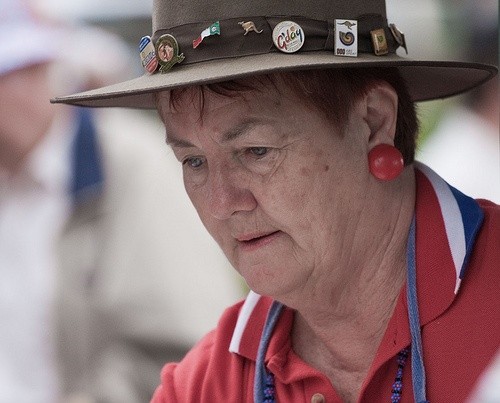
48;0;499;105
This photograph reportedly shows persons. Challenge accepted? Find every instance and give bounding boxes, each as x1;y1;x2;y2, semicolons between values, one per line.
409;2;498;202
48;0;500;401
0;0;182;402
